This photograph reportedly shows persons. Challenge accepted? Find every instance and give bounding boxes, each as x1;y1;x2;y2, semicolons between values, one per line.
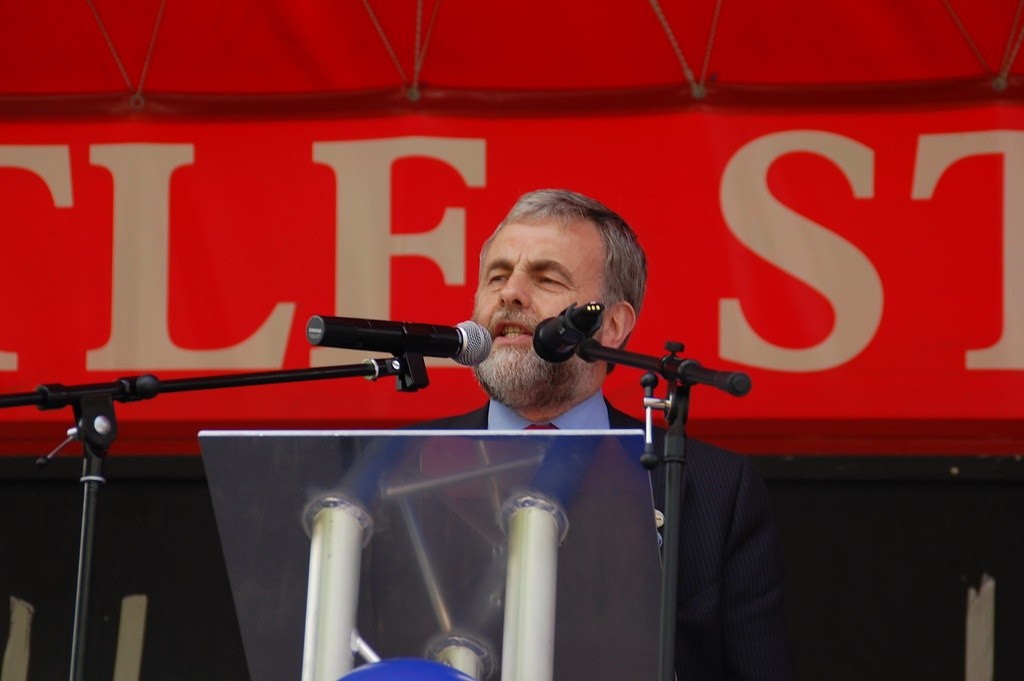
262;188;800;681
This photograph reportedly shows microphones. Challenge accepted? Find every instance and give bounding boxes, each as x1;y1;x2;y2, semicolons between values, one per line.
306;313;493;365
533;301;606;363
